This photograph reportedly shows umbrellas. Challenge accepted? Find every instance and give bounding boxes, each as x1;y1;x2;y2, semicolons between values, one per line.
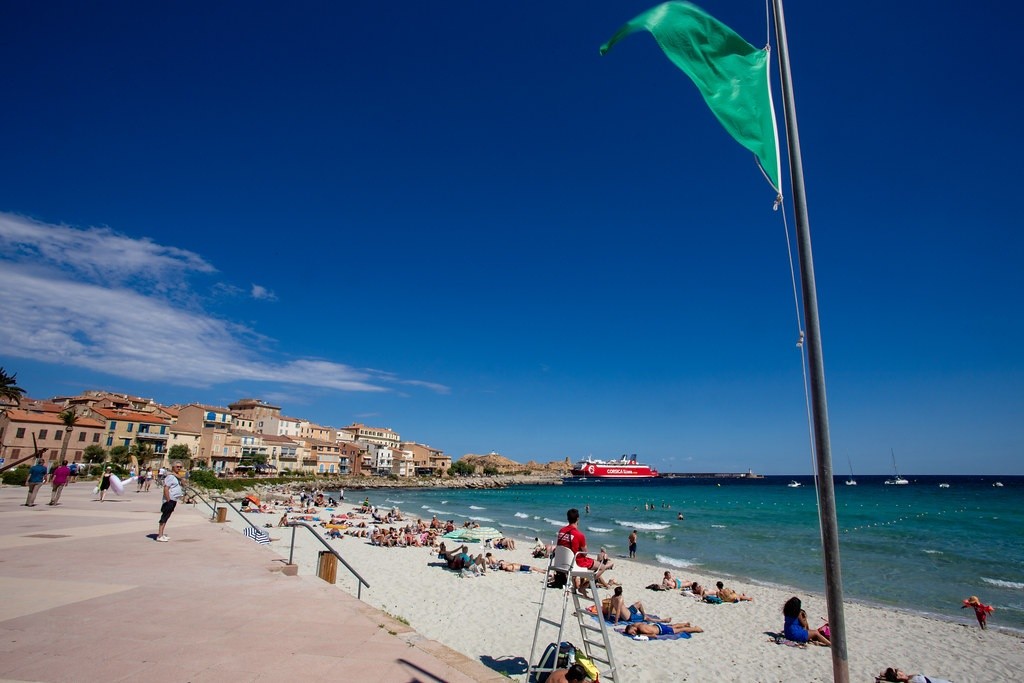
244;525;270;544
460;527;503;572
444;529;481;548
246;496;260;507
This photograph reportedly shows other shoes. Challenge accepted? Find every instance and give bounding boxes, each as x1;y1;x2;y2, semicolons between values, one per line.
157;537;168;542
24;503;28;506
29;504;36;507
163;535;170;540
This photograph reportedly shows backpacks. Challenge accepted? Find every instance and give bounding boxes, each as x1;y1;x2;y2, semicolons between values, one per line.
818;623;830;640
529;641;577;683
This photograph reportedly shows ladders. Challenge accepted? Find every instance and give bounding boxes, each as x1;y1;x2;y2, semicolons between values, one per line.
524;548;620;683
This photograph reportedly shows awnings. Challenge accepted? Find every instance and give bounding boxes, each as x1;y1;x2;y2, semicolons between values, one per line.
212;456;239;462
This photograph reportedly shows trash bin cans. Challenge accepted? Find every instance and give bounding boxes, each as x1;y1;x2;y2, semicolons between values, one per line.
316;550;338;584
217;507;227;523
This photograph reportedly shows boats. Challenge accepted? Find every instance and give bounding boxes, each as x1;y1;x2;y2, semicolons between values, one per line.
991;480;1006;488
787;480;805;488
570;454;664;478
937;482;952;488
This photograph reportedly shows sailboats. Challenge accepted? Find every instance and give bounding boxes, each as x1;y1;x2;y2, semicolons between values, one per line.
883;447;911;485
844;452;858;486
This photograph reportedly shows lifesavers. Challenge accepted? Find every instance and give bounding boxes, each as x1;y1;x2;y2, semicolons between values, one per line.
108;473;141;497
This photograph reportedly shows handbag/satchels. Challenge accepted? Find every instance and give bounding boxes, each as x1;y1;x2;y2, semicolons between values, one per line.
93;486;99;494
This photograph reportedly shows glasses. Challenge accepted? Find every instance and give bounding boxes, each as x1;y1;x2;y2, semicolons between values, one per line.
175;466;182;468
143;467;146;468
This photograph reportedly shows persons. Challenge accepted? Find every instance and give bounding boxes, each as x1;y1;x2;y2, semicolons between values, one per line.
625;618;703;638
463;522;478;528
783;597;831;647
607;586;650;625
49;460;70;505
24;459;47;506
597;546;608;565
716;581;751;601
240;487;455;547
878;668;950;683
961;596;994;629
557;509;605;598
645;502;683;519
69;462;79;482
157;460;183;543
438;538;545;576
662;571;693;588
595;576;616;587
586;504;589;514
628;530;638;557
97;465;168;502
534;537;555;558
692;582;716;595
